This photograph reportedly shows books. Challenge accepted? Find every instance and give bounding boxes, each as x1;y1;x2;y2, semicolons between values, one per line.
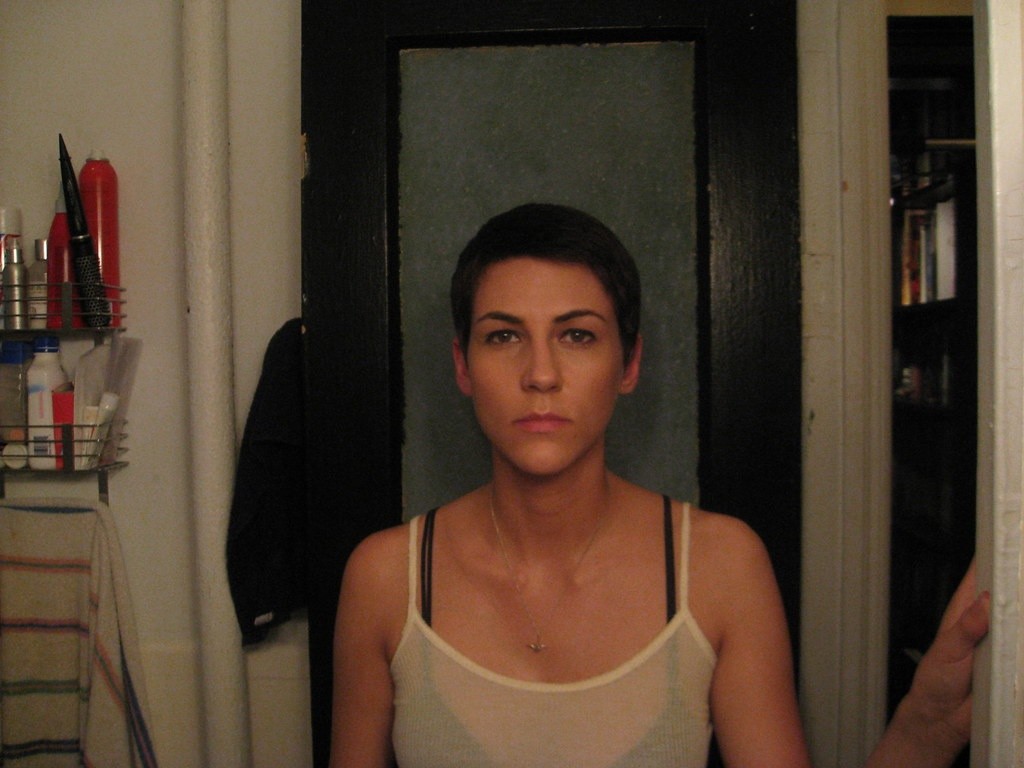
886;134;977;310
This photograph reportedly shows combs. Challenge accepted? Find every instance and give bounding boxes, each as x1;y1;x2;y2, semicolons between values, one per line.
59;133;112;327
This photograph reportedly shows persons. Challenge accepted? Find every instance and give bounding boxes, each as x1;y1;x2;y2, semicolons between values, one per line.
328;203;994;768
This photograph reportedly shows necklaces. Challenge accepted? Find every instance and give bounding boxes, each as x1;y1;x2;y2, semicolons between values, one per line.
490;490;600;651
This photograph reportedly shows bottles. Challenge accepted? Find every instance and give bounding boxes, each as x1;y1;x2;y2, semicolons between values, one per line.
71;334;112;470
25;336;67;469
0;340;32;444
